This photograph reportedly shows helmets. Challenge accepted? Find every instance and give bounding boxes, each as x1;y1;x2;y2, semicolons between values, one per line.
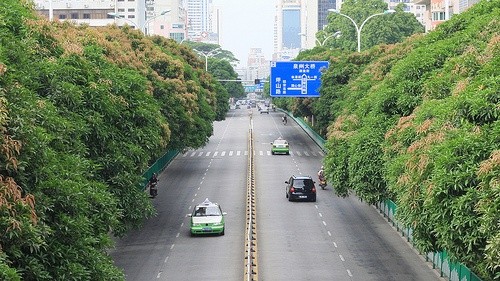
321;166;324;170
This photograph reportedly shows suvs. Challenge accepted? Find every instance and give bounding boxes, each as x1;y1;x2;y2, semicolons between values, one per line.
284;175;317;202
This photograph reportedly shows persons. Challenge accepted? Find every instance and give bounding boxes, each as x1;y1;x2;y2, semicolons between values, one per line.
149;173;158;195
196;208;205;216
283;115;287;123
318;166;326;181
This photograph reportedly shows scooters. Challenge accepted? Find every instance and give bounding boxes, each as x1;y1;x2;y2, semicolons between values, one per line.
283;120;287;125
147;179;160;198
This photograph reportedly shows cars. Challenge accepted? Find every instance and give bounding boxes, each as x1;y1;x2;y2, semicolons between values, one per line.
186;198;227;236
270;137;290;155
234;99;277;114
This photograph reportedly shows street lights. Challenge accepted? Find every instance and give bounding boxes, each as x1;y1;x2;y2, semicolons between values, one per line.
192;48;222;71
107;8;173;31
327;8;396;52
297;30;342;47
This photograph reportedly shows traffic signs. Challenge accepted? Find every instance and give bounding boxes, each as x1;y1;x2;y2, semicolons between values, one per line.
269;60;328;98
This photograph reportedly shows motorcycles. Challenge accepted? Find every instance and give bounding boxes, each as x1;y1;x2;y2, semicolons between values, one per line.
319;175;328;189
